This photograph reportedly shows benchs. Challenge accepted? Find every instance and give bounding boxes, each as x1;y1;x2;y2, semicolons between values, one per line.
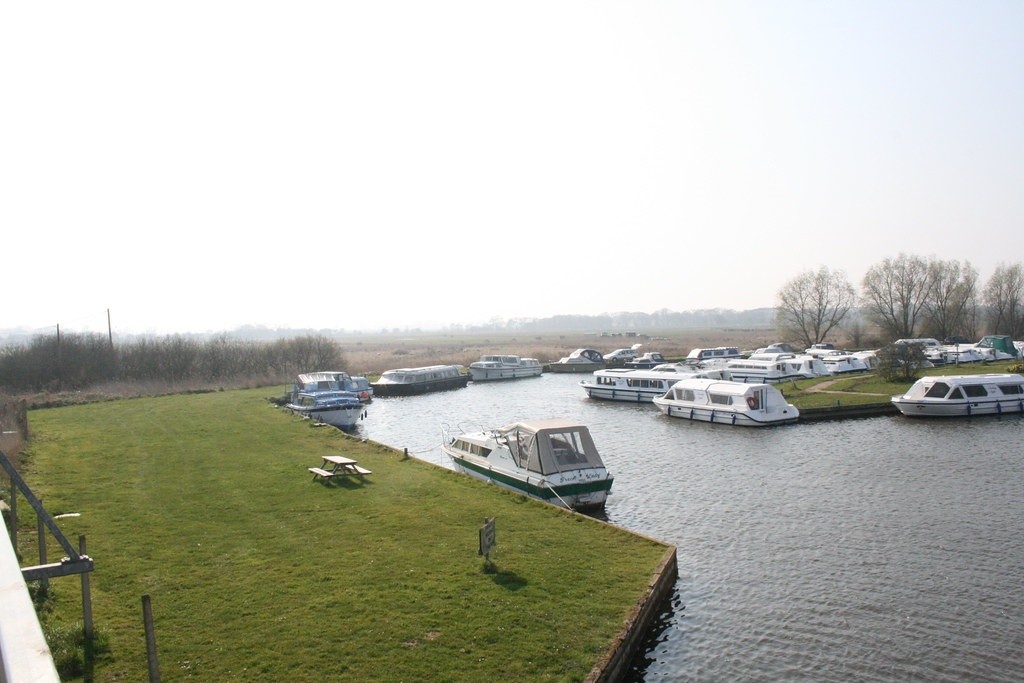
347;465;372;475
308;468;334;477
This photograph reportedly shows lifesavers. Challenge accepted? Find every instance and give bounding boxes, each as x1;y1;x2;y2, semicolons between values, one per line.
747;397;756;409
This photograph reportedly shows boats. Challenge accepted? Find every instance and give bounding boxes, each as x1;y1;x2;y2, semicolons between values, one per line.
651;377;799;428
350;376;376;403
891;374;1024;418
442;419;614;511
286;371;366;435
547;336;1024;405
367;364;475;397
469;355;543;382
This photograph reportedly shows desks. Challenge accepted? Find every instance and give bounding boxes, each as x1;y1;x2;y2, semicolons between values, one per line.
315;455;364;484
